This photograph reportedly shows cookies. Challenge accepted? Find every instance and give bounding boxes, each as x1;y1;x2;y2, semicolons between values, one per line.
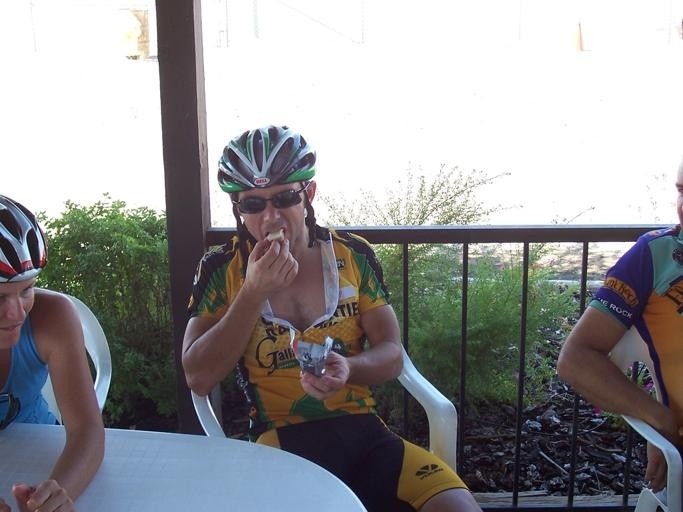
268;230;284;244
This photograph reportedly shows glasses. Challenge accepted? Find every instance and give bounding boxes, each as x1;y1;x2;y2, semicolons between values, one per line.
1;393;22;429
231;180;311;215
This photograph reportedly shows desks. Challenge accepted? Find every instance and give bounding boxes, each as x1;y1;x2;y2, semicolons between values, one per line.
0;419;370;512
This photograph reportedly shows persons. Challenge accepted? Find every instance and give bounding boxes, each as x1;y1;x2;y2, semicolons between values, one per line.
0;195;105;512
557;163;683;493
181;126;482;512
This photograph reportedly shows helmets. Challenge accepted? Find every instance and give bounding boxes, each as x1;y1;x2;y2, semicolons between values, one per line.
216;121;316;193
1;195;47;284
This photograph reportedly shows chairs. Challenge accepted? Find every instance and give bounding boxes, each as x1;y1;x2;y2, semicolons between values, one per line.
621;409;683;512
189;325;460;480
29;285;115;424
